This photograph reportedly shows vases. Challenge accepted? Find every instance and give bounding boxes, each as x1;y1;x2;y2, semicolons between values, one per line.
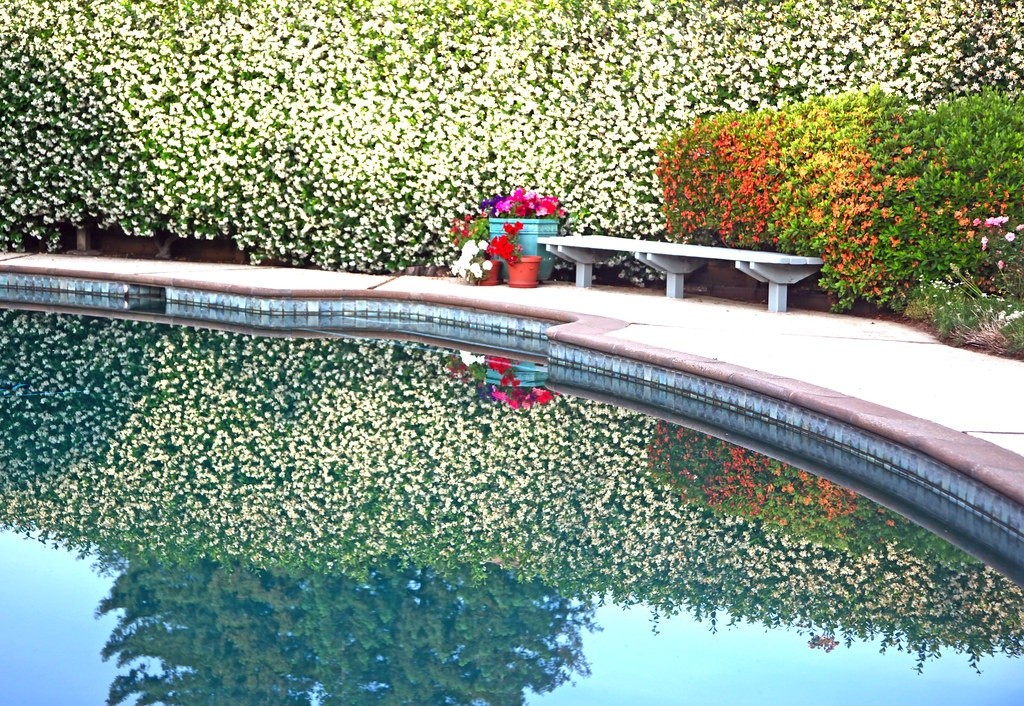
475;261;502;285
489;218;560;282
505;256;542;288
487;366;549;388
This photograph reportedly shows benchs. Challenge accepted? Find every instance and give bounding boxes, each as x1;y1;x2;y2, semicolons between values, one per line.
536;235;824;312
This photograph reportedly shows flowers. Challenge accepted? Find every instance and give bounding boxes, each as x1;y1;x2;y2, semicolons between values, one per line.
442;349;557;409
478;187;565;219
448;214;494;284
488;222;526;267
